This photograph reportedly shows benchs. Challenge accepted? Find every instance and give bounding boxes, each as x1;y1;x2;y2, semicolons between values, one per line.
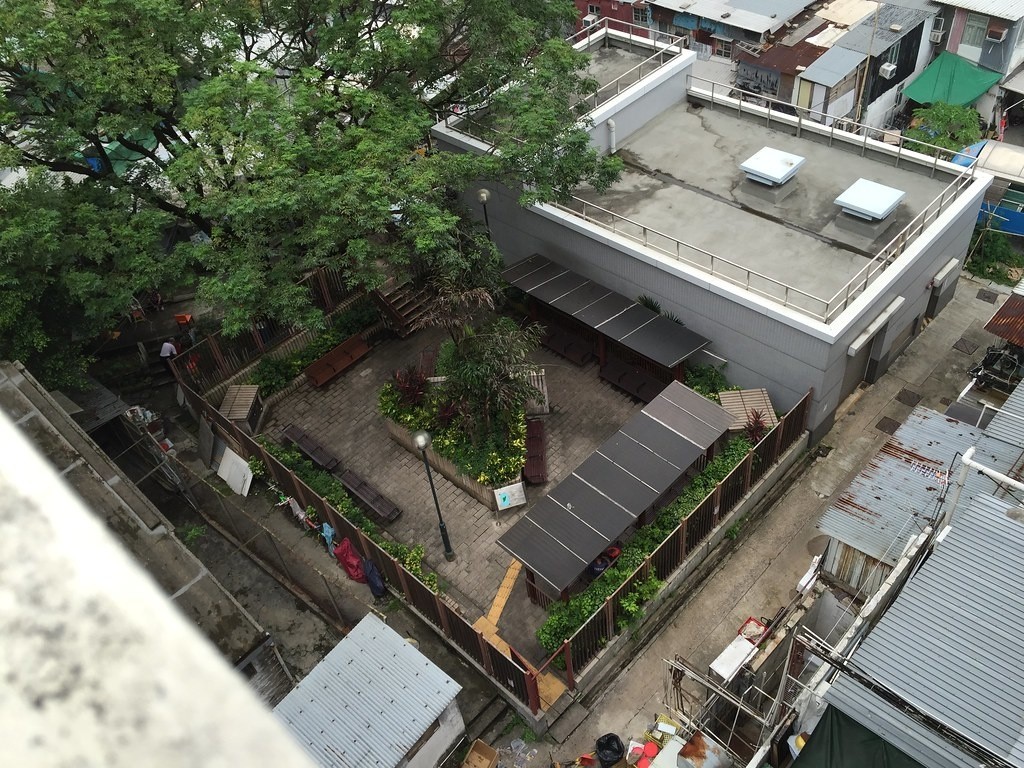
599;357;667;404
331;469;402;525
420;341;438;378
277;423;341;472
527;316;595;366
523;414;548;484
304;333;372;388
526;369;549;414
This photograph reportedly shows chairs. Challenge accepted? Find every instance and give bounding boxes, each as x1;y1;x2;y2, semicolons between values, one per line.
123;296;146;323
174;314;194;331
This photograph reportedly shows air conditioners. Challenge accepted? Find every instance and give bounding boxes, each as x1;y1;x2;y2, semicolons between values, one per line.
879;62;897;80
836;116;853;132
986;24;1008;42
929;30;946;44
582;14;598;30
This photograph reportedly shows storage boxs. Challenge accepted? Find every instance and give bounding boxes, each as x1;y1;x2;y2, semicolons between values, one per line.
631;713;680;768
460;738;500;768
737;616;772;646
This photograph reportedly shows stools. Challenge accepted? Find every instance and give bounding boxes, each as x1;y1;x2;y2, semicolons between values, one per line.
131;309;145;325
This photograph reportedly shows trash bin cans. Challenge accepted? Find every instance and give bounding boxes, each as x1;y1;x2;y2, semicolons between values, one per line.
595;733;625;768
152;430;165;441
592;558;607;575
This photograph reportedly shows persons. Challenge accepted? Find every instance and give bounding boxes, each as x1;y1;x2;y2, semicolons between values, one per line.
159;338;177;379
180;328;196;364
151;291;165;311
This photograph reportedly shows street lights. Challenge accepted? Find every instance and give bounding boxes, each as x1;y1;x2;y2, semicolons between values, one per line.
474;188;496;264
409;429;457;562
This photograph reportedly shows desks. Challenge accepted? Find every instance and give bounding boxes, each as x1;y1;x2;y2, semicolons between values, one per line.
795;555;822;597
